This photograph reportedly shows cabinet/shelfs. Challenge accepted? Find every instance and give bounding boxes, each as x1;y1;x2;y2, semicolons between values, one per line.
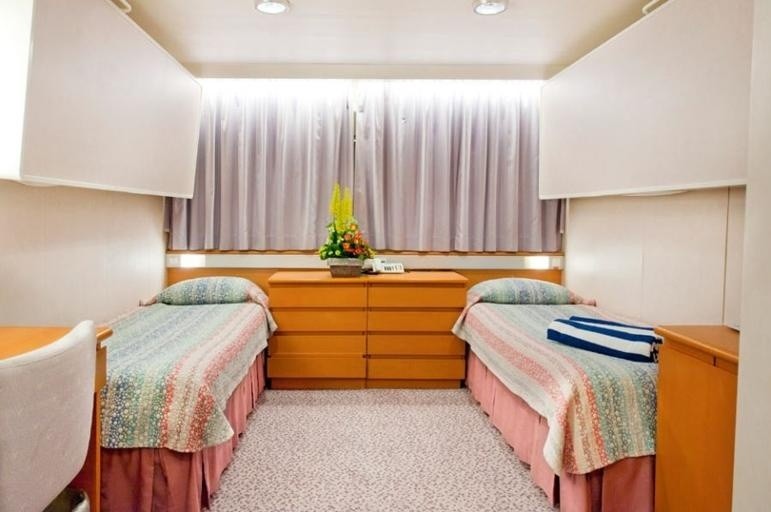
655;322;739;511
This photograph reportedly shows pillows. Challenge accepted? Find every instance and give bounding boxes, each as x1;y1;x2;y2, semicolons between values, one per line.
141;275;264;305
467;275;597;305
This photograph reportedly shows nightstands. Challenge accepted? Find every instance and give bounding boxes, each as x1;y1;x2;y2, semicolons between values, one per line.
368;271;469;388
267;270;368;388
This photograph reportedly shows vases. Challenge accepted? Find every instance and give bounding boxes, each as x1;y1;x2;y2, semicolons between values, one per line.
328;258;362;278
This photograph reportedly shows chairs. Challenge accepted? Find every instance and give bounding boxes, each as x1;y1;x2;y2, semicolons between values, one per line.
2;319;99;511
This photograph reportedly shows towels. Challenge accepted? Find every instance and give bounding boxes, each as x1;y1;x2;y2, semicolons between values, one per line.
546;315;664;362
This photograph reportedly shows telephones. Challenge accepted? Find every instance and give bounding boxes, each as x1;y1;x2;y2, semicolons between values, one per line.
371;257;404;274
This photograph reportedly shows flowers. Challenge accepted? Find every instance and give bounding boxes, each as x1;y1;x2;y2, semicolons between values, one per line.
318;182;377;259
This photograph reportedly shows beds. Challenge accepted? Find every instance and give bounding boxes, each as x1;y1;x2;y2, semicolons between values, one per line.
449;267;660;511
70;265;278;512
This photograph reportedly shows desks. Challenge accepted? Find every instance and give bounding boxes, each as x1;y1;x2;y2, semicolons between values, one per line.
1;325;116;511
